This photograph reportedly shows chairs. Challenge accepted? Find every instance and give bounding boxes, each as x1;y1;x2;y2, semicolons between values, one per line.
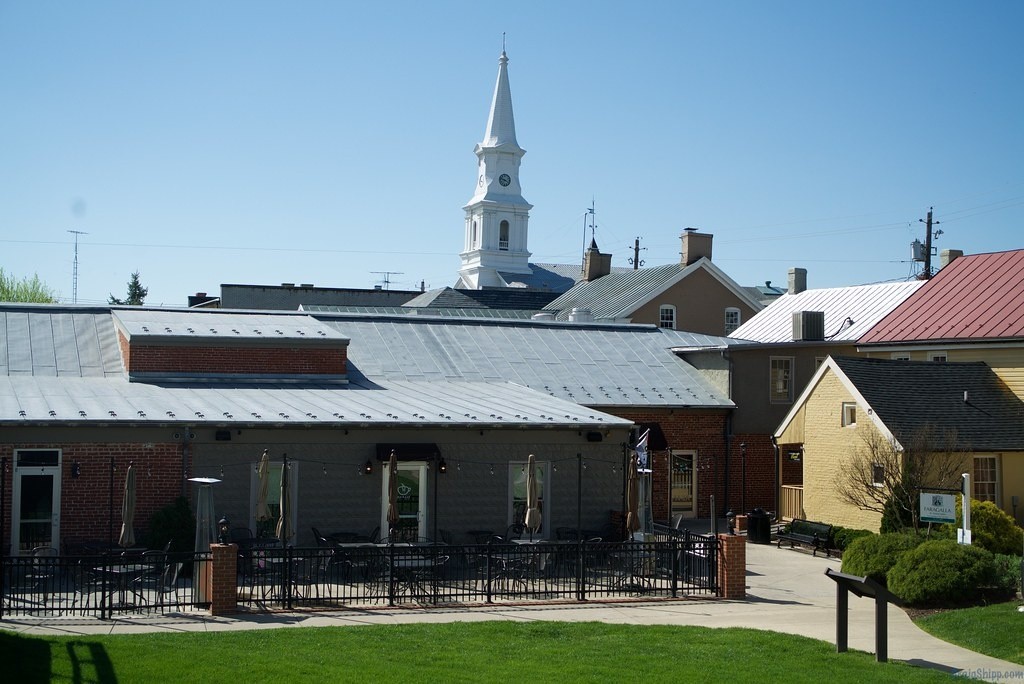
0;520;692;620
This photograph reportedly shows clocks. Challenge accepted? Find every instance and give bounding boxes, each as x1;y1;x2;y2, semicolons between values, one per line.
499;174;511;187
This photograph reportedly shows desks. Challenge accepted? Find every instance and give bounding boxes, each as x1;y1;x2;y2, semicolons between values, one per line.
259;557;305;599
608;553;654;587
480;553;532;593
386;556;437;603
94;564;154;607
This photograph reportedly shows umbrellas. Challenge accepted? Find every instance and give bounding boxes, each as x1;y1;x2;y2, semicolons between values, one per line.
525;455;541;541
194;477;217;609
118;461;136;566
387;449;400;541
276;459;295;544
627;452;641;584
257;449;272;534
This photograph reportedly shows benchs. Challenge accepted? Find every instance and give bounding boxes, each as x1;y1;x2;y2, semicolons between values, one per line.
777;518;833;557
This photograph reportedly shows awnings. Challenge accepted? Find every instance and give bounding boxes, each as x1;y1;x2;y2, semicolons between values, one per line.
376;443;442;461
629;423;668;451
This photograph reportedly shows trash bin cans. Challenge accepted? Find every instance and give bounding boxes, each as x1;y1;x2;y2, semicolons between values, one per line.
745;509;773;544
